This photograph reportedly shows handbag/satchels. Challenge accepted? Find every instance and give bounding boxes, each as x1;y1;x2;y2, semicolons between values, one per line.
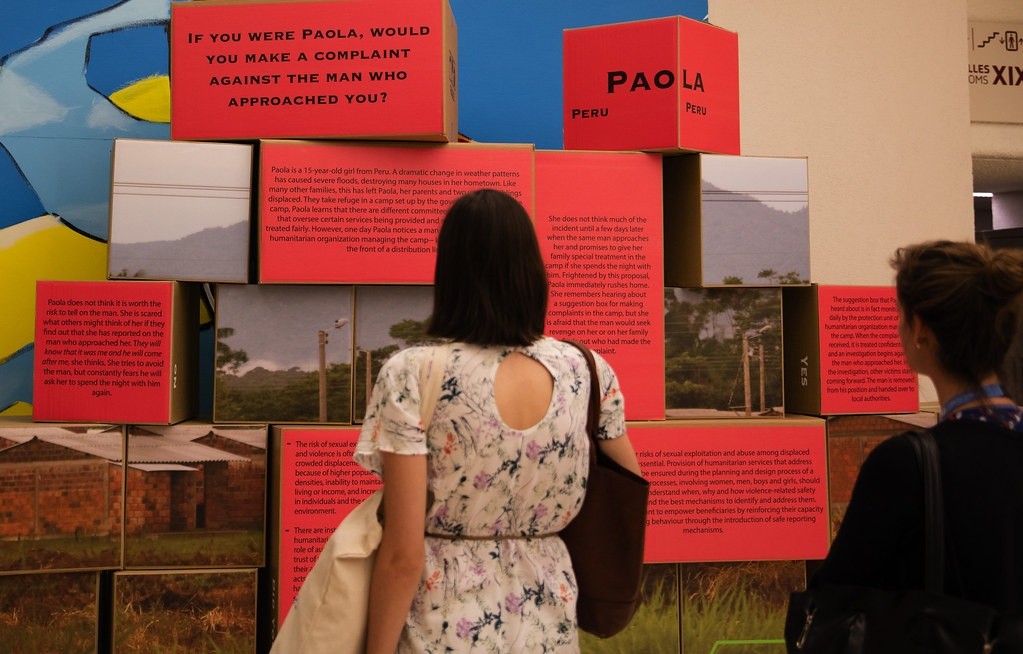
784;427;1023;654
268;344;446;654
557;339;651;639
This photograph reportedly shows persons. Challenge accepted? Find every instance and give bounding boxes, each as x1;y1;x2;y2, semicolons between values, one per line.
354;184;649;654
759;238;1023;653
